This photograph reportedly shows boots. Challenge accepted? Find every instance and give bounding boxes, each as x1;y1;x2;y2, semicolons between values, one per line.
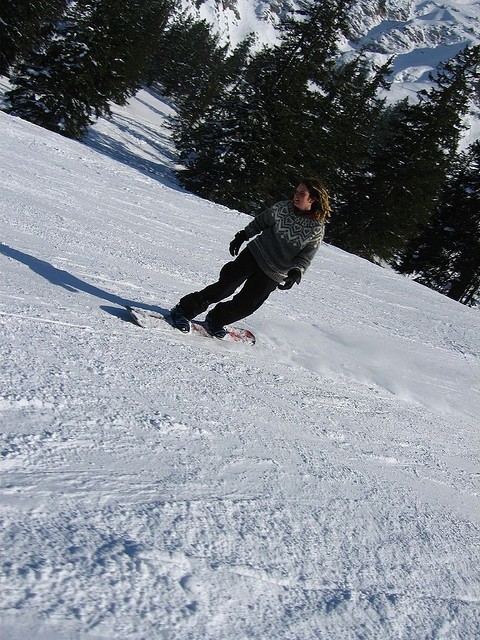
205;302;232;340
170;291;203;334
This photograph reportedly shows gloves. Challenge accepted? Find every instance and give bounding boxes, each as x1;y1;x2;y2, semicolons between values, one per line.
229;230;249;257
277;268;302;290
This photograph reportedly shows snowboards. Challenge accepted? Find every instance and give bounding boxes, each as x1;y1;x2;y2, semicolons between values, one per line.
124;303;256;347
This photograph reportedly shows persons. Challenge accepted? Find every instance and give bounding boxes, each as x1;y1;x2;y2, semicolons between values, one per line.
171;179;333;340
